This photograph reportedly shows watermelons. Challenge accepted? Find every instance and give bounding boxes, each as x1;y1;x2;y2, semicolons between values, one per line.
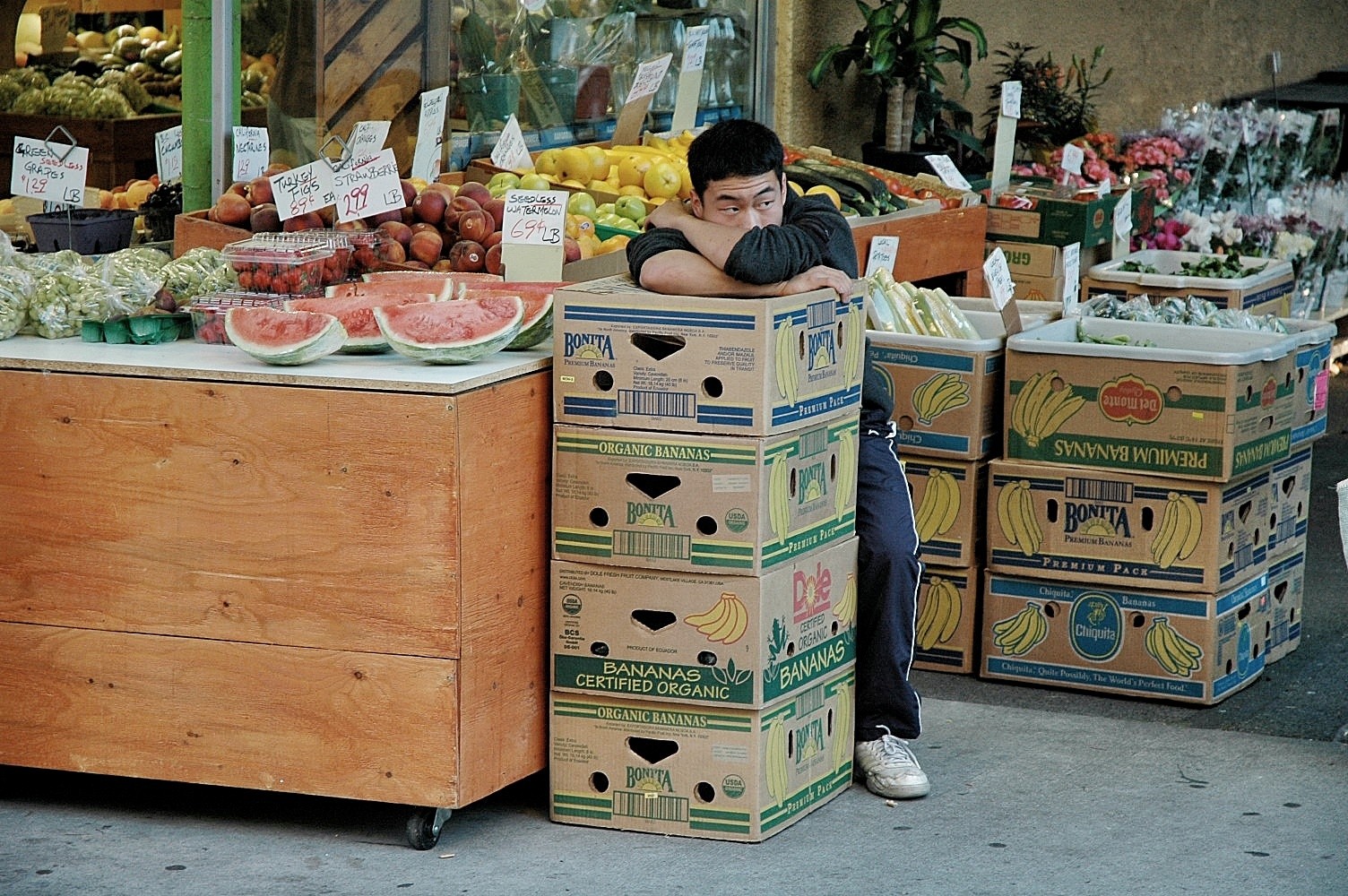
224;270;581;366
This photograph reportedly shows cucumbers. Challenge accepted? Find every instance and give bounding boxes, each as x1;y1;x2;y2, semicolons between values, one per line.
784;157;908;218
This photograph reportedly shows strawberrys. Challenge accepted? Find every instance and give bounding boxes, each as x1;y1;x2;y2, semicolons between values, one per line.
193;234;389;343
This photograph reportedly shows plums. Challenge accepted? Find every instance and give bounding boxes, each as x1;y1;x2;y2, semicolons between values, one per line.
137;182;183;242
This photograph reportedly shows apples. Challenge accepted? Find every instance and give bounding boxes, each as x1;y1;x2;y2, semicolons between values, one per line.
484;173;648;259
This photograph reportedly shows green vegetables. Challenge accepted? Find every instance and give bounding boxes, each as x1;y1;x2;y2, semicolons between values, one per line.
1117;253;1269;279
1082;294;1290;333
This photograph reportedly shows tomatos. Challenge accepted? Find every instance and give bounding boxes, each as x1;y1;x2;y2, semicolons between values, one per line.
783;145;962;209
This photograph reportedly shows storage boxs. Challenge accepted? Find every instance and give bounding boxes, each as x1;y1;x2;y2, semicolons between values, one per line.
973;178;1157;300
1081;252;1299;320
548;269;872;848
859;283;1336;705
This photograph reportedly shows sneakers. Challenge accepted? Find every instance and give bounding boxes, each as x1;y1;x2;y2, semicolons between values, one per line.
854;724;930;797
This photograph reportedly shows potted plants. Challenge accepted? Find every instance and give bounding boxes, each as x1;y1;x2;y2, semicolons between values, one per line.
806;0;994;176
982;38;1111;160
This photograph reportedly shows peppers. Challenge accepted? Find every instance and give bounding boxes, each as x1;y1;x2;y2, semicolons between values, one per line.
1076;321;1158;348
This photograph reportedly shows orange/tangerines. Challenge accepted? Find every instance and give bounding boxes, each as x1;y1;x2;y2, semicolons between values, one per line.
535;146;694;204
788;181;805;197
805;185;841;211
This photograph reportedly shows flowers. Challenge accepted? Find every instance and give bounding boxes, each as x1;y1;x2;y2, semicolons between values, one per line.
1011;100;1348;266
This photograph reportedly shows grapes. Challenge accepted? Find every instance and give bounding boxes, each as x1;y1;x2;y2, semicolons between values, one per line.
0;230;234;340
0;68;150;118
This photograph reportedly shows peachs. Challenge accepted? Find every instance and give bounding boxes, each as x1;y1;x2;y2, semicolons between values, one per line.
0;174;159;242
208;163;582;275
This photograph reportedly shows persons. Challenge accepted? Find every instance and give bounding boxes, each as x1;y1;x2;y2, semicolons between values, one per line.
626;119;931;799
